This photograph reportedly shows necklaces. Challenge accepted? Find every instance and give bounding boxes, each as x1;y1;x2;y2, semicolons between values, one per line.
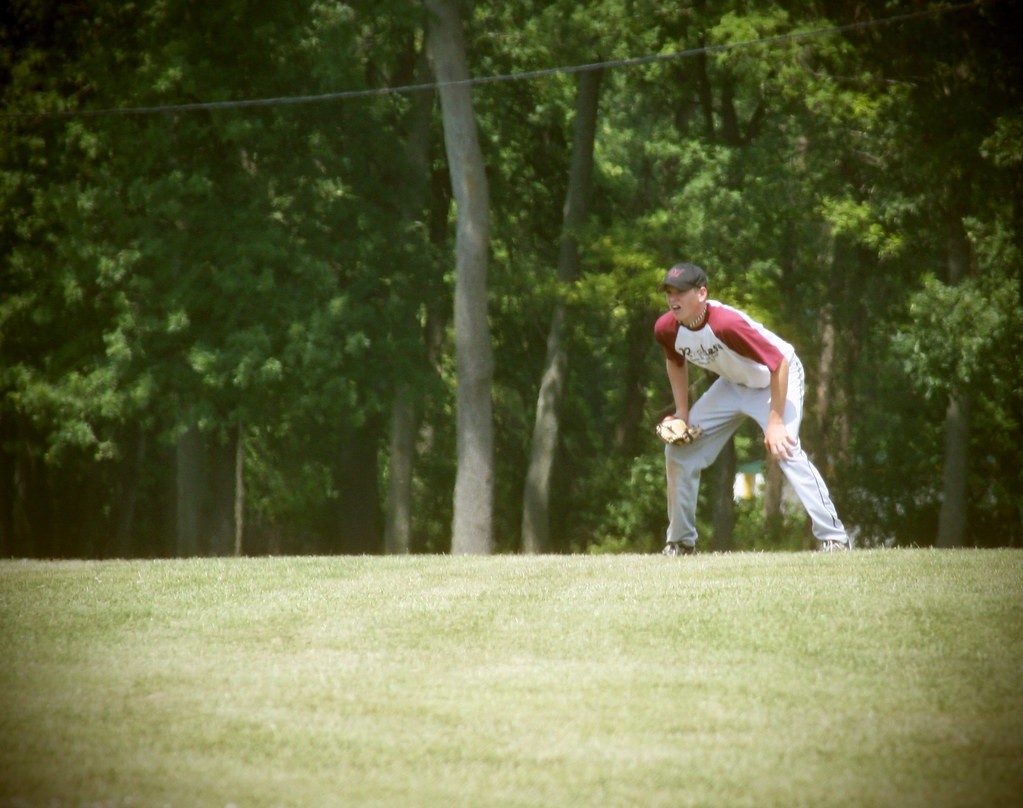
685;304;708;328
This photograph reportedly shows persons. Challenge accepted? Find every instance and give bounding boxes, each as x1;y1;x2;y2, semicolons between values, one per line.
653;261;852;556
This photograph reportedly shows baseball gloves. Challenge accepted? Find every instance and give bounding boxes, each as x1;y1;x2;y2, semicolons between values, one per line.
654;416;707;444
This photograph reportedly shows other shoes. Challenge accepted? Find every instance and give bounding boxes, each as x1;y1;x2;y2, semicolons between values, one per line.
661;541;696;557
818;531;852;553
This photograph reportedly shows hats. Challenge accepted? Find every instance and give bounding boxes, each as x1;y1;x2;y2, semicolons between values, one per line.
662;263;708;291
